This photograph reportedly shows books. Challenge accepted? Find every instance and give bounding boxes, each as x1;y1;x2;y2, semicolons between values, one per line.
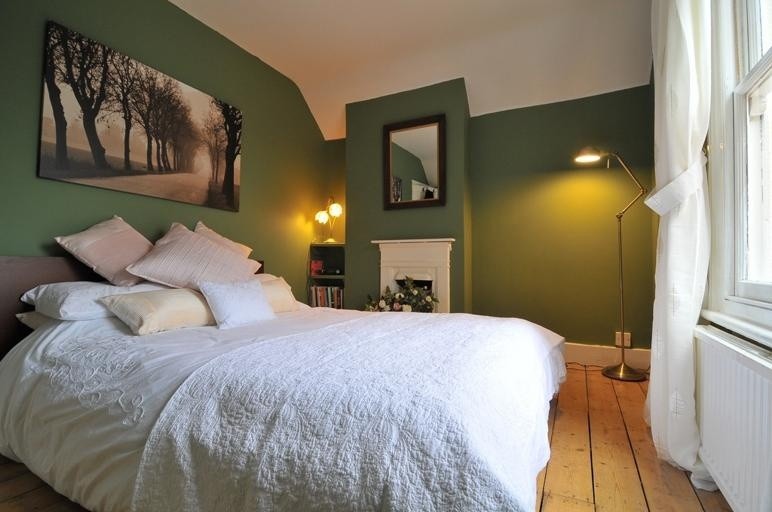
310;286;343;309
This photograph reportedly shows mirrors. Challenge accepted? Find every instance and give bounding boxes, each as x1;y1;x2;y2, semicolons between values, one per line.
381;110;447;209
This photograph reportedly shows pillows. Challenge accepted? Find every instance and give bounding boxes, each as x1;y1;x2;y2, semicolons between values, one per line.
12;214;302;336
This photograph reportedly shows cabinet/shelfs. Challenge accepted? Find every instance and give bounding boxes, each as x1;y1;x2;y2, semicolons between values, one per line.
309;242;347;309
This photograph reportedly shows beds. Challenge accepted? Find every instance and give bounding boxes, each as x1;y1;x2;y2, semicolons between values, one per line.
2;239;571;512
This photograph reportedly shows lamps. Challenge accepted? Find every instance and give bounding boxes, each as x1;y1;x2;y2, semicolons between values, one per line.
573;144;648;384
314;194;343;243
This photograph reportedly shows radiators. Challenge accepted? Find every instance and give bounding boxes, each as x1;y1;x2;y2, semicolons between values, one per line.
689;323;772;512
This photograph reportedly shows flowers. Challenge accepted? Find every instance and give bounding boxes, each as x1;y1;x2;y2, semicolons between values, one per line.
361;275;440;312
